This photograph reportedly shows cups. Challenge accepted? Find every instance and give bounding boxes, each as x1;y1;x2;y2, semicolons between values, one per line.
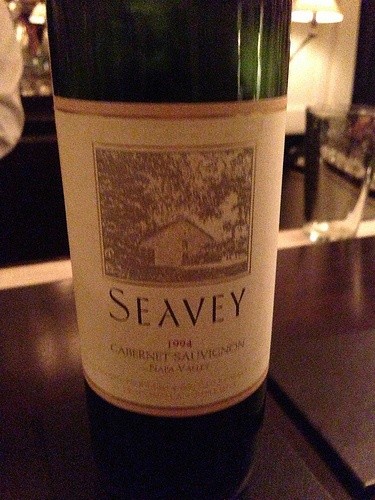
304;104;374;240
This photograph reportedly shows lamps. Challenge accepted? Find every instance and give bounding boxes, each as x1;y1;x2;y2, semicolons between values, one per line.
290;0;342;63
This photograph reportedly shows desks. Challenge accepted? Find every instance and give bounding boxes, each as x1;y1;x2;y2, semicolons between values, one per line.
1;237;375;500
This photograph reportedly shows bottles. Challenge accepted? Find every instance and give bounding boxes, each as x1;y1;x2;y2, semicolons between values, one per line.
44;0;291;500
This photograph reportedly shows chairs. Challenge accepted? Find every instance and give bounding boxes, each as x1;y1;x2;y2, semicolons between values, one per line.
282;107;330;219
